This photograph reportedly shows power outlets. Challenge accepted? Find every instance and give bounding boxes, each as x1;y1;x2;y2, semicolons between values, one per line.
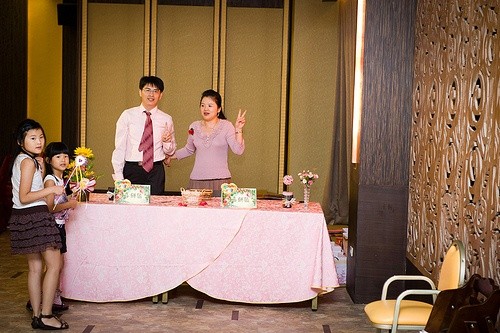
350;246;353;256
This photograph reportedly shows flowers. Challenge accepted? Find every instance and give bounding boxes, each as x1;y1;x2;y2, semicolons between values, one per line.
298;169;319;186
65;147;98;182
282;175;293;192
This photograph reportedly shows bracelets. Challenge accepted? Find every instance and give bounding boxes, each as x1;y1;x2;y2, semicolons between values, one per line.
235;131;243;135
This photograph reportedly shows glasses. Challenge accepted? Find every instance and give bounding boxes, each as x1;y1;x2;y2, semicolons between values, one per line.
143;88;160;94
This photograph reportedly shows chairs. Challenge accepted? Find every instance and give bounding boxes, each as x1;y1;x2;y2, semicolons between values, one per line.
364;239;500;333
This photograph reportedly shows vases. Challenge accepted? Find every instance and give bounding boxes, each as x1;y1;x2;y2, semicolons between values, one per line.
76;190;90;201
304;186;310;207
283;192;293;207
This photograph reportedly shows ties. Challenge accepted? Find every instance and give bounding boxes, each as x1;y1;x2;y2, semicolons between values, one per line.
138;112;153;173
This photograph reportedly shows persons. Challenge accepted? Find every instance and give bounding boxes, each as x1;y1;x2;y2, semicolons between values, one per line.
8;119;69;329
163;89;247;197
111;76;177;196
26;142;78;311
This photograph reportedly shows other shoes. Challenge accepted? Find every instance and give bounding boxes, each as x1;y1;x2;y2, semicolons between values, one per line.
31;314;69;330
52;303;68;310
27;300;34;311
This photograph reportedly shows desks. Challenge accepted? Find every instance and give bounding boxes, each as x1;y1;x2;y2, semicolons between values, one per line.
59;193;339;311
